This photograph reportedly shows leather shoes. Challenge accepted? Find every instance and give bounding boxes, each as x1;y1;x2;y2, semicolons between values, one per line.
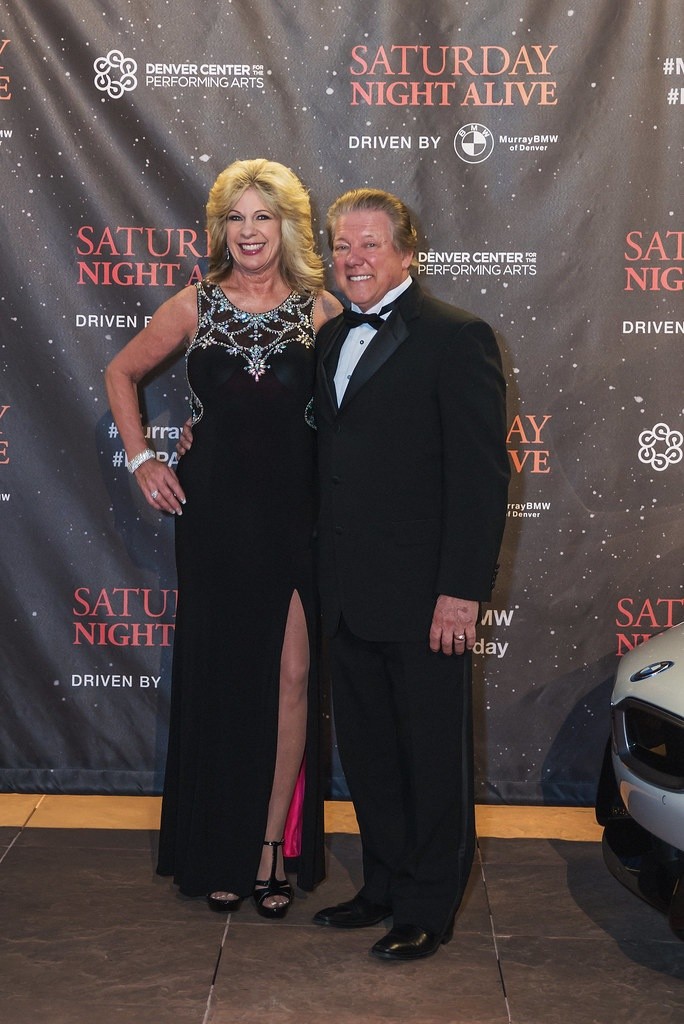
312;897;396;929
371;923;454;961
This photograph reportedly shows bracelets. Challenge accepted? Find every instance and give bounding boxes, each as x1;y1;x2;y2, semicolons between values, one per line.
126;449;155;474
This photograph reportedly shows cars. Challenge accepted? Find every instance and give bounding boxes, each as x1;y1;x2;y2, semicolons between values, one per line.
609;621;684;852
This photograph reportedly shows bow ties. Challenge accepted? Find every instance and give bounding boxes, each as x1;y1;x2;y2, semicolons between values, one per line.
343;307;385;333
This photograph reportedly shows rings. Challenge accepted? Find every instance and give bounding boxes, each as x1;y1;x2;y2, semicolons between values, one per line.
151;490;160;499
455;634;465;640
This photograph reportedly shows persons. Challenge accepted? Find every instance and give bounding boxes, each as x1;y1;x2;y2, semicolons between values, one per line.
103;160;350;922
173;189;509;962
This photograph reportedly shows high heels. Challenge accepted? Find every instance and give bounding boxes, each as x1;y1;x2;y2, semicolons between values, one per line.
206;888;241;911
255;840;294;918
